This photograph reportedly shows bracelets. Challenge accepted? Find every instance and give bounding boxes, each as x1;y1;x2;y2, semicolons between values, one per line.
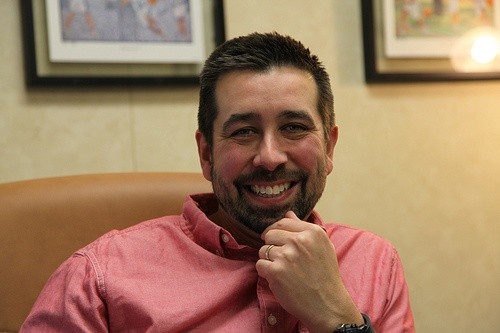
333;311;378;333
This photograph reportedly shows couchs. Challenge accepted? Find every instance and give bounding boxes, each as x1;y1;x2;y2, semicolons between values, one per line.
0;172;213;333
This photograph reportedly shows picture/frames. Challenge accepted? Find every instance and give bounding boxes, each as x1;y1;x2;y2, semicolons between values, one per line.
20;0;227;87
361;0;500;83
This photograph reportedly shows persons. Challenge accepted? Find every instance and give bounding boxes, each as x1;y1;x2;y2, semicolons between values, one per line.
19;29;418;333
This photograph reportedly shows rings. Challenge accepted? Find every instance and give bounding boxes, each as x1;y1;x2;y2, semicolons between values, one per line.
264;243;275;260
